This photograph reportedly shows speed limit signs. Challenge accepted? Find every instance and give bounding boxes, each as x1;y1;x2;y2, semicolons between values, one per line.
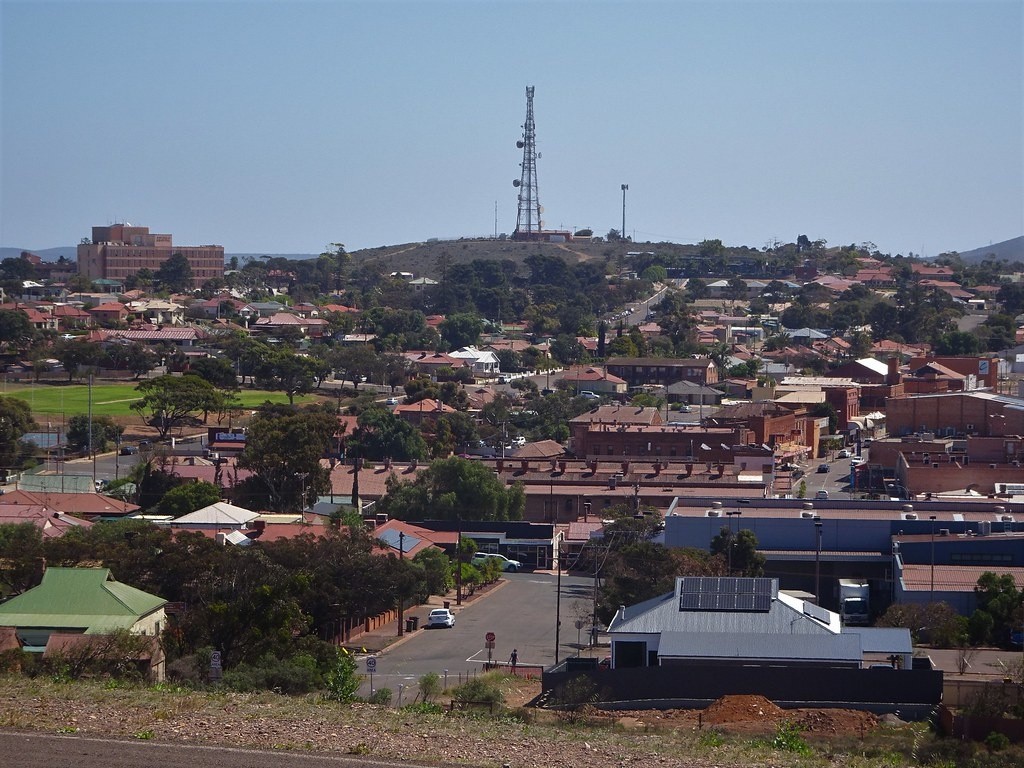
366;657;376;673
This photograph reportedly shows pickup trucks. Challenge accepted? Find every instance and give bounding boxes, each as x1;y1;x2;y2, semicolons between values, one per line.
474;554;520;572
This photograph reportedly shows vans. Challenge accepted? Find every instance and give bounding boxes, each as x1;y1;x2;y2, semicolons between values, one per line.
864;438;874;447
541;390;555;396
498;376;511;384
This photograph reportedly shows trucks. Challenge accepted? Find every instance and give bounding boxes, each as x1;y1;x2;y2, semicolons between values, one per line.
837;578;870;624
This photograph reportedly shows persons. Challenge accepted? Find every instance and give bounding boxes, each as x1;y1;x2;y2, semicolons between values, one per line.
508;648;521;675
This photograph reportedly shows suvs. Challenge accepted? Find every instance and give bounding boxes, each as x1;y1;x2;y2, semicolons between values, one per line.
581;391;600;400
139;441;152;451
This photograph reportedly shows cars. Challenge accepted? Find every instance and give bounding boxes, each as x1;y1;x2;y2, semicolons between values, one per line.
817;464;830;473
838;450;851;458
386;398;398;405
121;447;139;455
471;552;488;560
863;664;894;669
428;608;456;628
680;406;692;413
816;490;829;499
851;456;865;466
598;655;612;672
